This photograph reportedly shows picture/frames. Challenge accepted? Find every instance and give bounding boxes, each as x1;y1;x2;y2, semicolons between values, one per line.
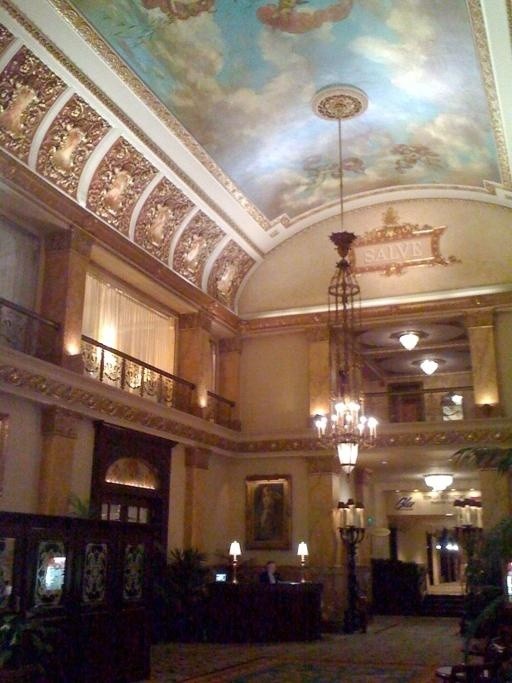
241;472;294;551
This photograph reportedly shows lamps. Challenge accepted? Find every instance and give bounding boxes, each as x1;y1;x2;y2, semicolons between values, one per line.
412;358;447;375
311;84;382;481
390;328;430;350
424;471;454;490
336;495;368;632
296;540;309;582
452;494;484;636
228;540;242;583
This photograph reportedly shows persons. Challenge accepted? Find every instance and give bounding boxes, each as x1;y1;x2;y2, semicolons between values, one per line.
257;561;283;583
476;404;496;417
415;405;425;420
255;485;280;537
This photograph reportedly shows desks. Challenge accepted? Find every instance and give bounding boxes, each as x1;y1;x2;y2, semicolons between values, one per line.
434;660;511;682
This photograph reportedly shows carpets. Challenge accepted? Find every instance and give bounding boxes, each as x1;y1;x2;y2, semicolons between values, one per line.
176;651;439;682
